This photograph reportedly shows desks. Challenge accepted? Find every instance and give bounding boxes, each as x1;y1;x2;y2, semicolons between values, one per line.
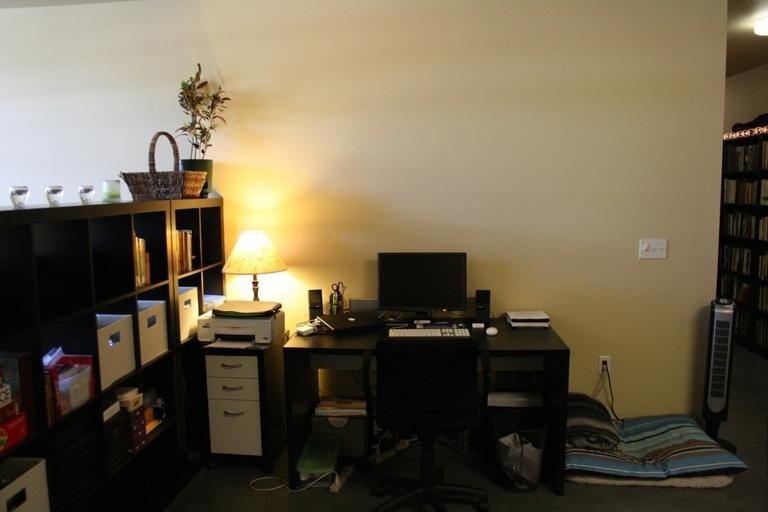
277;307;572;495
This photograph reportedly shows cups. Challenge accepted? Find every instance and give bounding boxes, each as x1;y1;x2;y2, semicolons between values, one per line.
46;184;65;205
181;170;207;199
99;180;123;204
9;186;29;206
78;184;95;204
329;302;343;316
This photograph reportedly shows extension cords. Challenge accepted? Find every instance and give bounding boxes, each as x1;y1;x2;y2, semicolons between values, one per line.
368;440;410;465
329;464;356;493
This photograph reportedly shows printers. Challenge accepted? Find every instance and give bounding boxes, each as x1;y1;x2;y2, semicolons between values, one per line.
198;301;284;351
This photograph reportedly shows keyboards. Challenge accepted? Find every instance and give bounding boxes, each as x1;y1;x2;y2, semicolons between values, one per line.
388;328;471;337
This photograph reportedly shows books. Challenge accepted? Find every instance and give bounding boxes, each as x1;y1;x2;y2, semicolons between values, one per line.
316;408;367;415
316;310;361;329
717;138;767;352
175;229;195;275
507;314;549;328
506;309;550;322
317;394;367;408
136;235;153;287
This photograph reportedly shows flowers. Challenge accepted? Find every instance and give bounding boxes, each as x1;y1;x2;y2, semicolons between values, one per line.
171;61;231;166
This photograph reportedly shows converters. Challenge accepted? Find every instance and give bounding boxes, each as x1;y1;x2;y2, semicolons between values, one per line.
296;323;317;336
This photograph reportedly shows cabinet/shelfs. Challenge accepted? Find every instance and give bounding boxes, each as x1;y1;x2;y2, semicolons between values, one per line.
202;323;295;473
0;193;226;512
715;114;768;359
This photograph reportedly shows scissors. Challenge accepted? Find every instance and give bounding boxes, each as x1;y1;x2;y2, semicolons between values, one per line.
332;282;343;294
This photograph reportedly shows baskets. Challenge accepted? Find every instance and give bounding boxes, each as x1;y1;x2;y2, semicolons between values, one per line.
119;130;209;202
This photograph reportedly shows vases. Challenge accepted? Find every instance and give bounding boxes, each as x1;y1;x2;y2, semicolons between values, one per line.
180;158;214;199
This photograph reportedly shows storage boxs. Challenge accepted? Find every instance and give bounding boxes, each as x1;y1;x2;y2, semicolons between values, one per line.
0;456;51;512
53;356;93;417
136;294;174;367
96;307;139;389
176;282;205;345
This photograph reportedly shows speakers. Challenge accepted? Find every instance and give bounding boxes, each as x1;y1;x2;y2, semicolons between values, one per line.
350;299;379;313
475;290;490;325
308;289;323;324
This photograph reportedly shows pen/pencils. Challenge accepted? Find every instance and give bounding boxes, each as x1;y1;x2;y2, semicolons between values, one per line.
329;292;343;315
316;316;334;331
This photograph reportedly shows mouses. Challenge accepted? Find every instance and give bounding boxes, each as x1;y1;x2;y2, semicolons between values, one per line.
486;327;498;336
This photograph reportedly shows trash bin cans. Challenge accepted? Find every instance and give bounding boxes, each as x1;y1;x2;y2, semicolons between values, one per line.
492;410;547;493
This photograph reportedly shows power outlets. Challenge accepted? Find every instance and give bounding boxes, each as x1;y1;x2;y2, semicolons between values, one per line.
598;355;610;376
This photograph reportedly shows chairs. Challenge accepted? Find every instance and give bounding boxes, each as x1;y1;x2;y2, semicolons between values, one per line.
357;333;494;512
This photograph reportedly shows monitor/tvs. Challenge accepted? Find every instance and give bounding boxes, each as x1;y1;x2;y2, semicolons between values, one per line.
377;252;467;324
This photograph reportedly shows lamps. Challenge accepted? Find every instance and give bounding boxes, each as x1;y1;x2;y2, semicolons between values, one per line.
222;230;289;302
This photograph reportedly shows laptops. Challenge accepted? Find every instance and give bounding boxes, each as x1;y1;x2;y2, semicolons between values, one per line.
317;308;386;333
